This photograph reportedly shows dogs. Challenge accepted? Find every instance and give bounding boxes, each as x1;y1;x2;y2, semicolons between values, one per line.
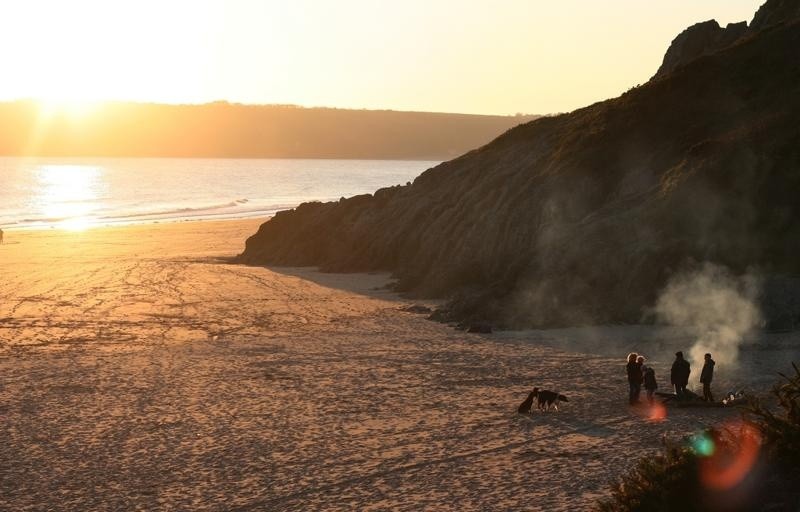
533;387;569;412
518;390;539;414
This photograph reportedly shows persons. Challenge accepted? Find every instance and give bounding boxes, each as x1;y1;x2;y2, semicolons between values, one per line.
640;365;657;403
670;352;690;394
626;353;641;406
700;354;715;402
637;356;645;403
